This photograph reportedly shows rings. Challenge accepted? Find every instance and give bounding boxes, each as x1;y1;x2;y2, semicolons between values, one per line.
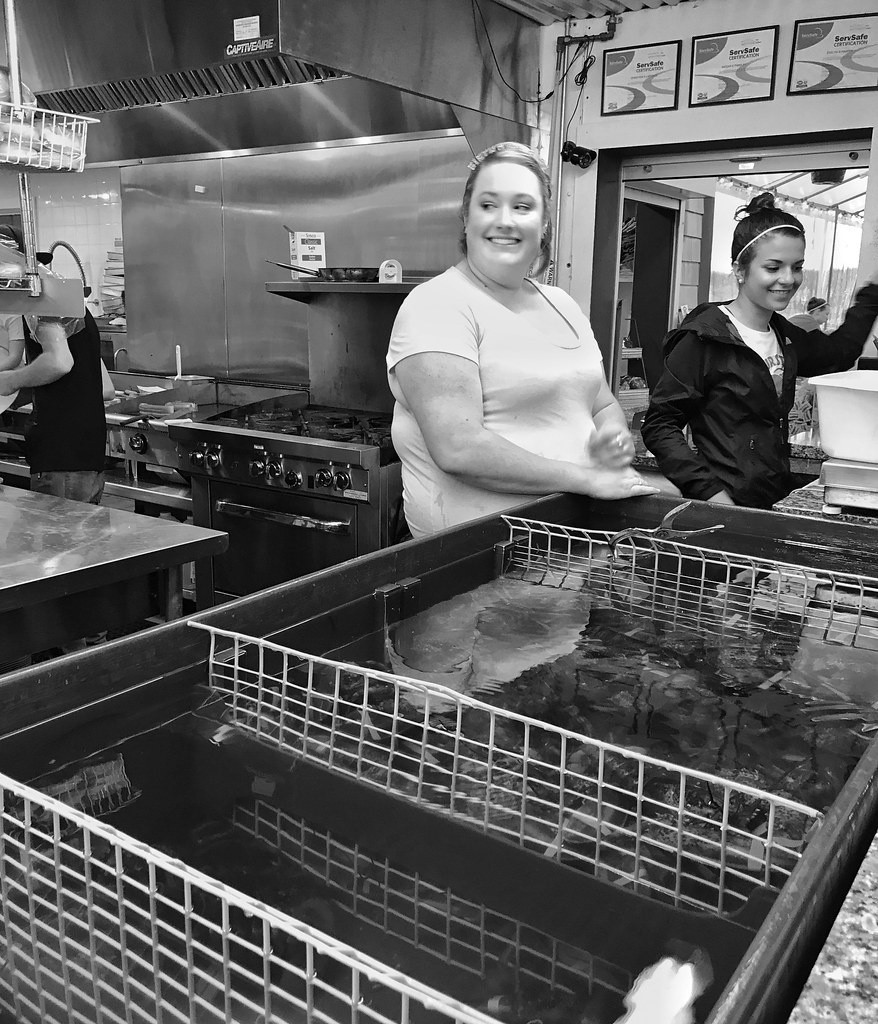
616;436;624;448
638;478;642;485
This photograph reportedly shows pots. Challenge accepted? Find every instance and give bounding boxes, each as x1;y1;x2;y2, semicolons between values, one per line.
264;257;379;282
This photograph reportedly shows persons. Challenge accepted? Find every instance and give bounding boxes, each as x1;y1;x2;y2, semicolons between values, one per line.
787;296;830;332
385;545;613;716
639;189;878;512
387;141;662;542
0;224;106;504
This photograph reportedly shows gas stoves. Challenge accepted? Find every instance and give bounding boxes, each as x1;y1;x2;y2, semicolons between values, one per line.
163;405;402;506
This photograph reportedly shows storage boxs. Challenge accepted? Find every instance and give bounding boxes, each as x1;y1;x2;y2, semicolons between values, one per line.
808;369;878;465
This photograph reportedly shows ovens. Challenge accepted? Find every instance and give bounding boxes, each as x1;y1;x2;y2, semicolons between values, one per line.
190;476;413;612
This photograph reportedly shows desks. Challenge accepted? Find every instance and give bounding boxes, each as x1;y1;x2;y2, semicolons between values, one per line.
0;482;231;675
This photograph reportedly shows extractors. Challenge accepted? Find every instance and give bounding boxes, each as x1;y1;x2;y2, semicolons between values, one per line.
265;270;447;307
